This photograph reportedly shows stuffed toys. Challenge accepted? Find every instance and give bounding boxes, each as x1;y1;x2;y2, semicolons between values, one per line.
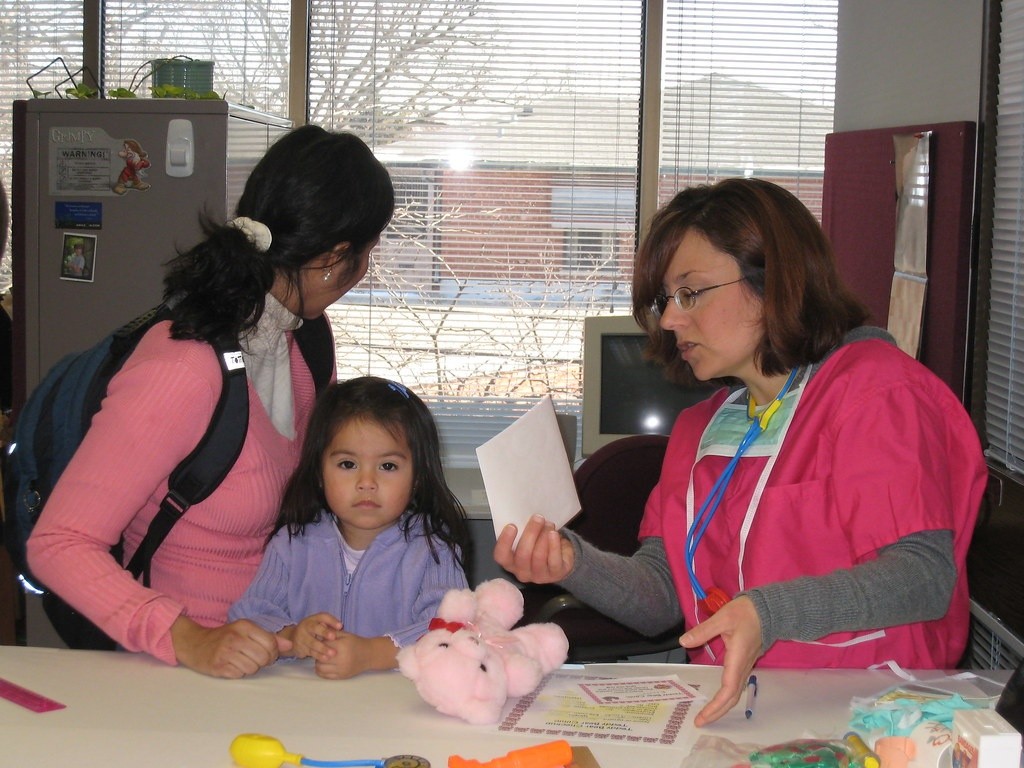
396;578;569;726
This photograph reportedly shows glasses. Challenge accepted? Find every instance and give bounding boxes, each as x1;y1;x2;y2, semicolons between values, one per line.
652;278;747;316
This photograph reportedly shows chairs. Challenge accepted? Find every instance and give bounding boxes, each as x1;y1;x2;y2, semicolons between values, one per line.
505;433;693;663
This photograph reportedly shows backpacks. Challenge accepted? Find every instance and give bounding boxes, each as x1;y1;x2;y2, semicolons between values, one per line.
3;310;335;651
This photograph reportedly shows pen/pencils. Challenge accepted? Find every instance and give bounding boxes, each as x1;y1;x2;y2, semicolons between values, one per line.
745;674;758;719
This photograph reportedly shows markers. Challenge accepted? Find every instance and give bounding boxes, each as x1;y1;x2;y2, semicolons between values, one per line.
844;731;881;768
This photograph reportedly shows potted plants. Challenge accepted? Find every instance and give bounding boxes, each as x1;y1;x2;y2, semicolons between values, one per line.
26;55;228;102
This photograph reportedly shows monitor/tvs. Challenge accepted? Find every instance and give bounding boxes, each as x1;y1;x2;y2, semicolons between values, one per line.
581;316;739;458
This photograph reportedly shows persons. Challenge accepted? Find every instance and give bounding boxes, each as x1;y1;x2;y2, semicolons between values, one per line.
25;126;395;679
223;377;470;674
65;244;85;276
492;179;986;726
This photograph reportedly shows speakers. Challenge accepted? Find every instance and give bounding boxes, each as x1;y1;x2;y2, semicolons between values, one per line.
556;414;577;475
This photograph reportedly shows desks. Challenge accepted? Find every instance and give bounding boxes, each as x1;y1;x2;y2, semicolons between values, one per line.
0;643;1024;768
